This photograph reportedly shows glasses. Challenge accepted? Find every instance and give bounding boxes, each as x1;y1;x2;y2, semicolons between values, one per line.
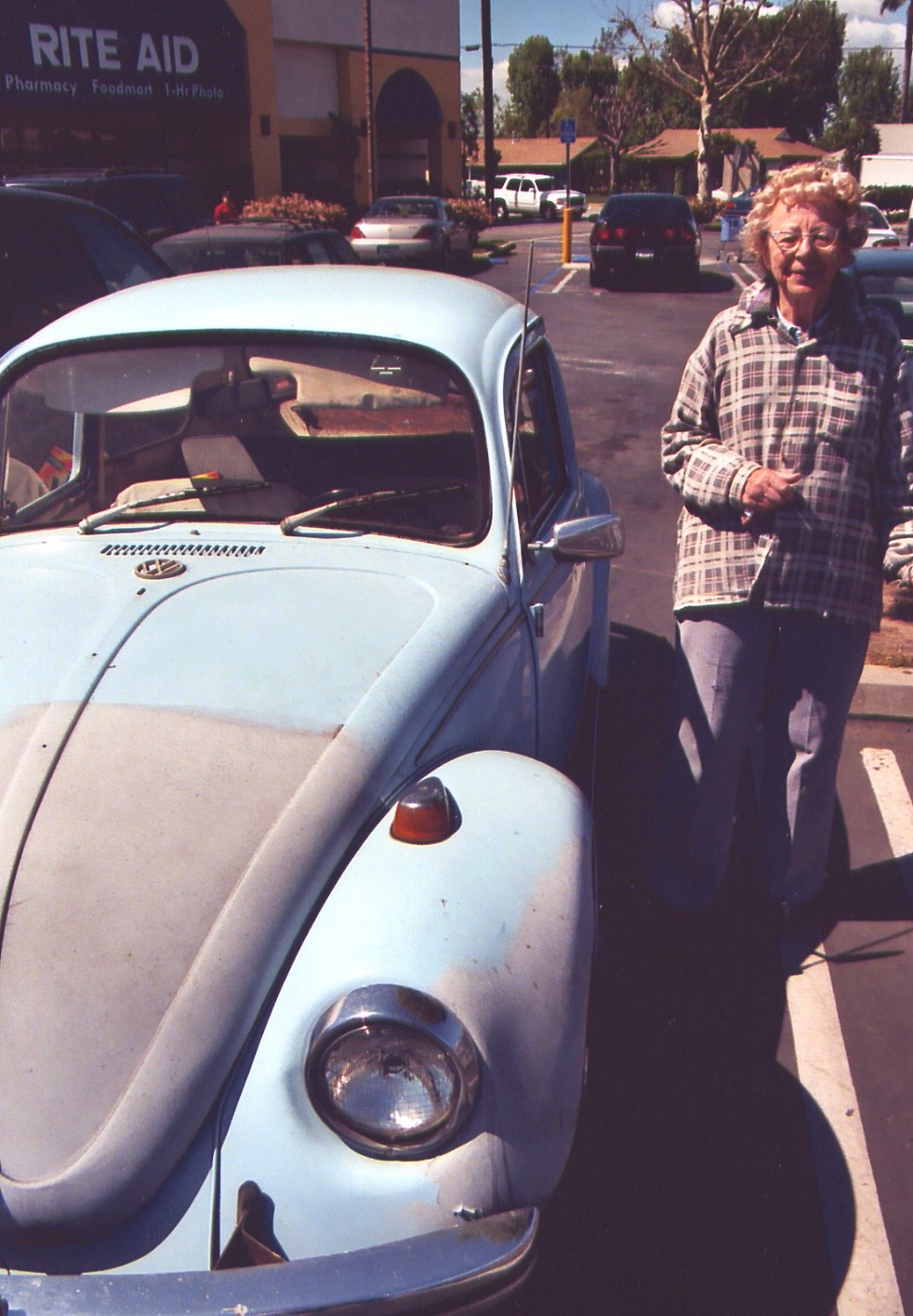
762;226;848;254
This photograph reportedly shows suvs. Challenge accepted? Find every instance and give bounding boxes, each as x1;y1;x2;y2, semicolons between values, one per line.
0;168;360;361
492;173;587;221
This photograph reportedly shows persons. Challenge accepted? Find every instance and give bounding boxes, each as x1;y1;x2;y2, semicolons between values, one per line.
658;162;913;945
214;191;240;226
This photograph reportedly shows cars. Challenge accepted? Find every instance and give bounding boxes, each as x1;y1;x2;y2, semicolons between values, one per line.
587;193;701;288
0;266;627;1316
720;185;902;295
350;195;476;268
